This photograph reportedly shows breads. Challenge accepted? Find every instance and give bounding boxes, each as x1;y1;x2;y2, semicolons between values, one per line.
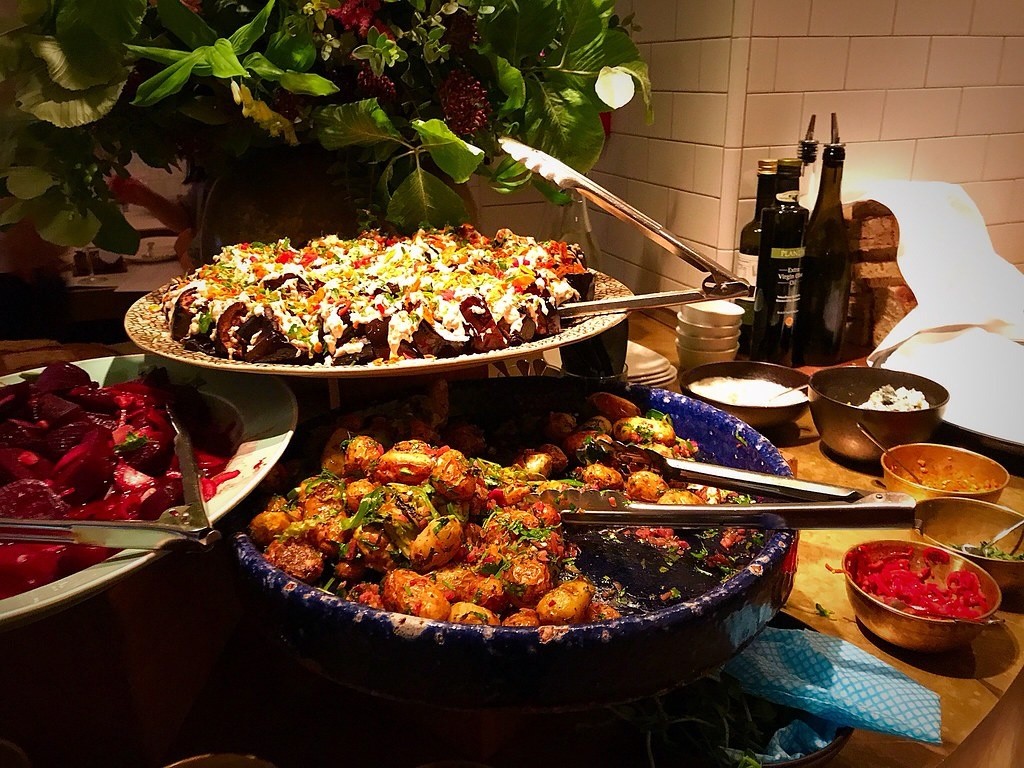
839;201;918;350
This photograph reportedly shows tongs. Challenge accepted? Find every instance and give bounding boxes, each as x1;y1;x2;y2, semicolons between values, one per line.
0;363;223;559
529;438;917;532
497;136;751;318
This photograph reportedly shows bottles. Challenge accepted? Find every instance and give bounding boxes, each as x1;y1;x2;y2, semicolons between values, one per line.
737;112;855;369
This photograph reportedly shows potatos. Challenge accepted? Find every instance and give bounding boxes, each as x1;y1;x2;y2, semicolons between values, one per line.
244;392;703;627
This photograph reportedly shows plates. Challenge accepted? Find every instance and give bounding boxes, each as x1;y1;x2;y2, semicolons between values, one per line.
124;267;636;380
542;340;678;389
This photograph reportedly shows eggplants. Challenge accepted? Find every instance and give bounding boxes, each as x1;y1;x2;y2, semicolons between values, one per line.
158;235;597;362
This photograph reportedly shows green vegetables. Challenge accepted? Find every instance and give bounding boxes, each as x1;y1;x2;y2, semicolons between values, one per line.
949;538;1024;561
272;410;762;621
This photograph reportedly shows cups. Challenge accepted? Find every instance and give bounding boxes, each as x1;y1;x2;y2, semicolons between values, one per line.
558;318;629;378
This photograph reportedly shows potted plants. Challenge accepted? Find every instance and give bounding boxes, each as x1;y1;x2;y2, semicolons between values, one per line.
1;0;659;263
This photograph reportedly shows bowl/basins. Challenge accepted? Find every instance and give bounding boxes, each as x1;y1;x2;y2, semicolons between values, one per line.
910;496;1024;589
674;299;746;369
881;443;1010;504
232;385;802;717
840;538;1002;650
0;354;298;631
678;361;811;430
807;365;951;460
